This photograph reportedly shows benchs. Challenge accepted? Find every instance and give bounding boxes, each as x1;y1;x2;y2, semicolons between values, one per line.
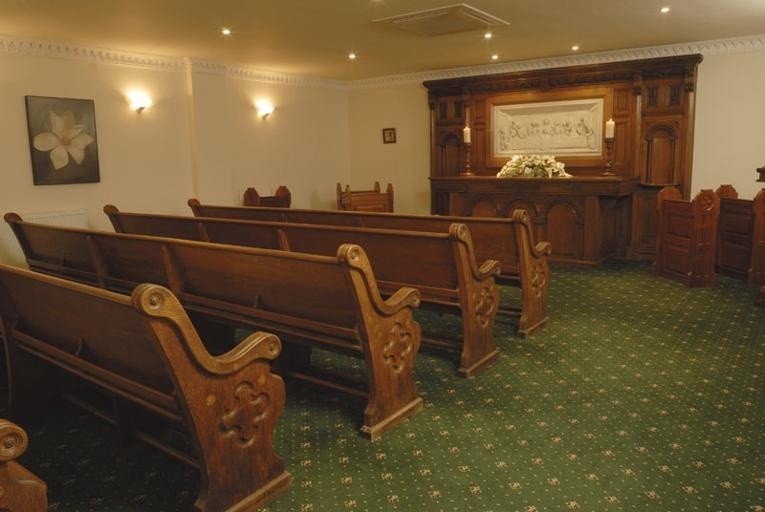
102;202;501;379
188;197;549;338
2;263;288;512
4;209;422;441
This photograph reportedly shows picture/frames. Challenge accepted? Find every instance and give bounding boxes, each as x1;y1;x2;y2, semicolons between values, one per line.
26;95;100;186
382;128;396;144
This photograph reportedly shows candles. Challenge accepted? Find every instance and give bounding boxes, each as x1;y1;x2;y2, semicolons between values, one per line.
463;125;471;143
606;117;615;139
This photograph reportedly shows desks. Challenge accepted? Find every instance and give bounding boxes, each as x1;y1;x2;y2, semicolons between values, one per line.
428;175;640;266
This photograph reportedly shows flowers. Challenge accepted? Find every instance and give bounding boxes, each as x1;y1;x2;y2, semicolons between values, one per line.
497;155;573;178
